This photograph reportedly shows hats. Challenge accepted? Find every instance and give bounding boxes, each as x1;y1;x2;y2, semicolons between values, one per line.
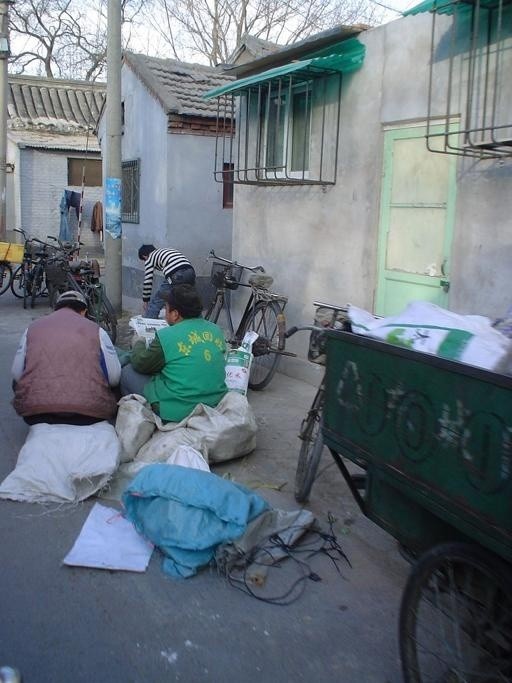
57;290;88;305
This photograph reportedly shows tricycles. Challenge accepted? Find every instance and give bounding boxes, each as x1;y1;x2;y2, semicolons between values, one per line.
293;298;512;683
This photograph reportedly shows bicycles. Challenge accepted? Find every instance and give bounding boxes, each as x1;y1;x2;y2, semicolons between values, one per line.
0;227;118;345
203;248;297;392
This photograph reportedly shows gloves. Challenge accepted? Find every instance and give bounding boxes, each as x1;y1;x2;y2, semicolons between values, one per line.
130;332;146;350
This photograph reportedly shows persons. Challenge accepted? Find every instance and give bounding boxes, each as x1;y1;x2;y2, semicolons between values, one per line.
116;281;229;427
137;243;197;319
10;289;123;426
